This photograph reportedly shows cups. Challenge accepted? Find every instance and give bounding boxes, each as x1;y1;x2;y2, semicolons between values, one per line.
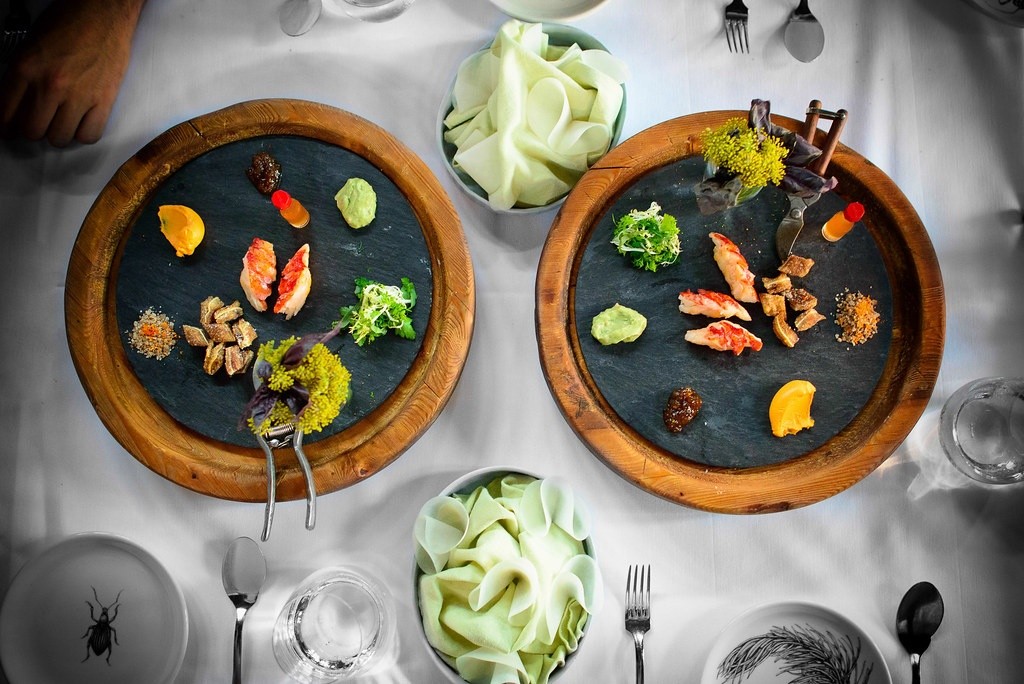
936;376;1024;485
270;563;398;684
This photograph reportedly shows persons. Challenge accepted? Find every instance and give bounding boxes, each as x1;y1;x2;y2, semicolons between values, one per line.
1;0;147;149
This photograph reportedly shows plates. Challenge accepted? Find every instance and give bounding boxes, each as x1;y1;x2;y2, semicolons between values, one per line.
534;108;949;518
967;0;1024;28
699;599;893;684
485;0;609;24
0;530;189;684
60;95;477;507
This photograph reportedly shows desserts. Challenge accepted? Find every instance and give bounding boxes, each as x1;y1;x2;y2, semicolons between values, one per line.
412;473;602;684
445;18;623;214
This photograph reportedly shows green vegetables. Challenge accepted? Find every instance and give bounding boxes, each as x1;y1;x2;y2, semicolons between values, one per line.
613;202;680;271
332;275;418;346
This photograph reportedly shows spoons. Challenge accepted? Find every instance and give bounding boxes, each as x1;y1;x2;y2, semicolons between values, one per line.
278;0;322;38
220;535;268;684
894;581;945;684
783;0;825;64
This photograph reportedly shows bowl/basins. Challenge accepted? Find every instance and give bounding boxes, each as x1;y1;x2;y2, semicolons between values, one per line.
434;22;628;216
407;463;597;684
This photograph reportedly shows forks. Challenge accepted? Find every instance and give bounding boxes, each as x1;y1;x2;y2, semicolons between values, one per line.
722;0;751;55
624;563;653;684
